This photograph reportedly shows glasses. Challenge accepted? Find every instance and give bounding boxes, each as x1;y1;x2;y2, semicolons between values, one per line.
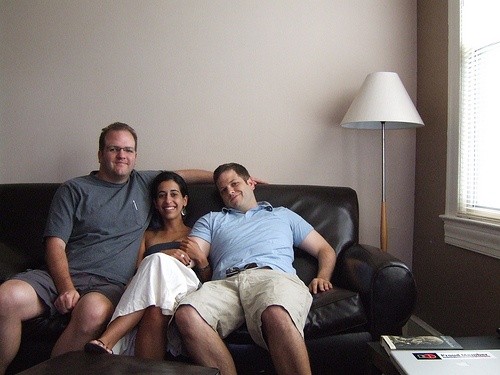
226;262;259;277
101;146;135;154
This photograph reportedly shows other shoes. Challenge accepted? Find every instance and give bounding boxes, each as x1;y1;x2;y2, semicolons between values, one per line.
84;338;113;354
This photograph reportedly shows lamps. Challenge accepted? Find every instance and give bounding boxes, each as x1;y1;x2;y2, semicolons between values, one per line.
340;71;425;251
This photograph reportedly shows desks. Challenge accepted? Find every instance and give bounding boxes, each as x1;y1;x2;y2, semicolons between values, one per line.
366;336;500;374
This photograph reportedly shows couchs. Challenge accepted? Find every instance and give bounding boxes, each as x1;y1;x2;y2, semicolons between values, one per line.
0;183;415;375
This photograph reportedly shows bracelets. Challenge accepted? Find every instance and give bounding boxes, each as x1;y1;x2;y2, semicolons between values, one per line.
196;263;210;272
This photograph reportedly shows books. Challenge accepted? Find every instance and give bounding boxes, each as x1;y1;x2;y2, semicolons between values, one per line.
380;335;500;375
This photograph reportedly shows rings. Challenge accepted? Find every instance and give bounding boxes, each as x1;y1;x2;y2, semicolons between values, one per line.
181;253;185;258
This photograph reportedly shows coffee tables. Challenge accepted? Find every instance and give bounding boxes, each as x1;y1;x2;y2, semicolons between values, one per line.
14;349;222;375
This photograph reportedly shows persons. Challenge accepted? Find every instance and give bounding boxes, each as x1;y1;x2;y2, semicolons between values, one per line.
0;122;272;375
165;163;338;375
87;171;213;359
